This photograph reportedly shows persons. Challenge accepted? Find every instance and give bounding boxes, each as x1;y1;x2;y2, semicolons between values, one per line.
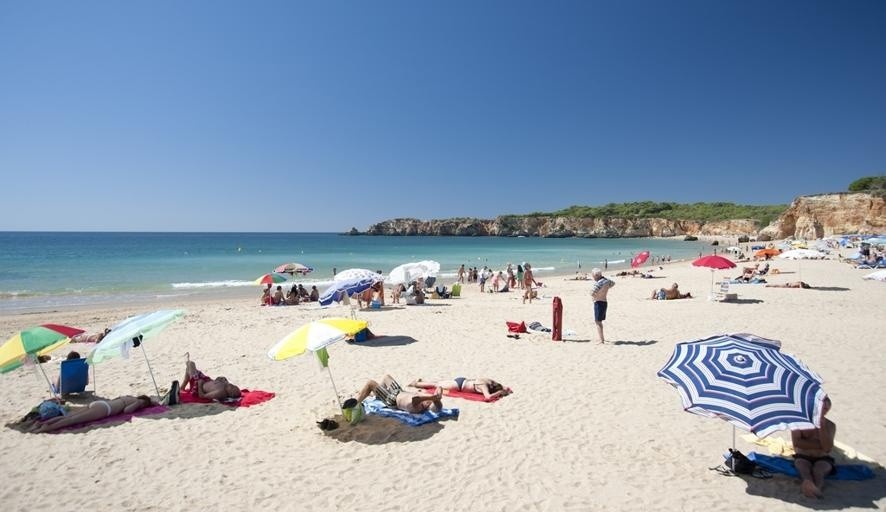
179;353;240;399
351;373;442;415
257;260;543;310
653;283;680;298
53;349;81;393
27;395;151;434
68;328;103;346
651;255;671;266
791;395;836;499
715;248;769;279
588;266;615;345
409;375;511;401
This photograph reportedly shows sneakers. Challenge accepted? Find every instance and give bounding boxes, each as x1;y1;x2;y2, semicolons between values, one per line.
320;419;338;430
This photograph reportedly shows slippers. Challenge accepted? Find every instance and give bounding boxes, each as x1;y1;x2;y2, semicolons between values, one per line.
753;465;773;478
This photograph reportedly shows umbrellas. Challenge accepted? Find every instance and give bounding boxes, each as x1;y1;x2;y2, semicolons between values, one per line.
264;316;368;418
83;308;186;401
1;321;83;415
657;332;827;473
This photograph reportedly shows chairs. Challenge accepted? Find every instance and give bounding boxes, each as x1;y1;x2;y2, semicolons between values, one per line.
56;359;98;399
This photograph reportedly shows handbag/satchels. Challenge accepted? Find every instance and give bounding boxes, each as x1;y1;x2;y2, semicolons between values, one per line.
724;448;755;475
341;401;365;424
506;321;526;332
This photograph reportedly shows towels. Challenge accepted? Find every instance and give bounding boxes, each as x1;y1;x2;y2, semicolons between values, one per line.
177;388;275;407
739;432;883;471
421;385;504;403
37;401;171;434
725;451;877;482
364;398;459;426
